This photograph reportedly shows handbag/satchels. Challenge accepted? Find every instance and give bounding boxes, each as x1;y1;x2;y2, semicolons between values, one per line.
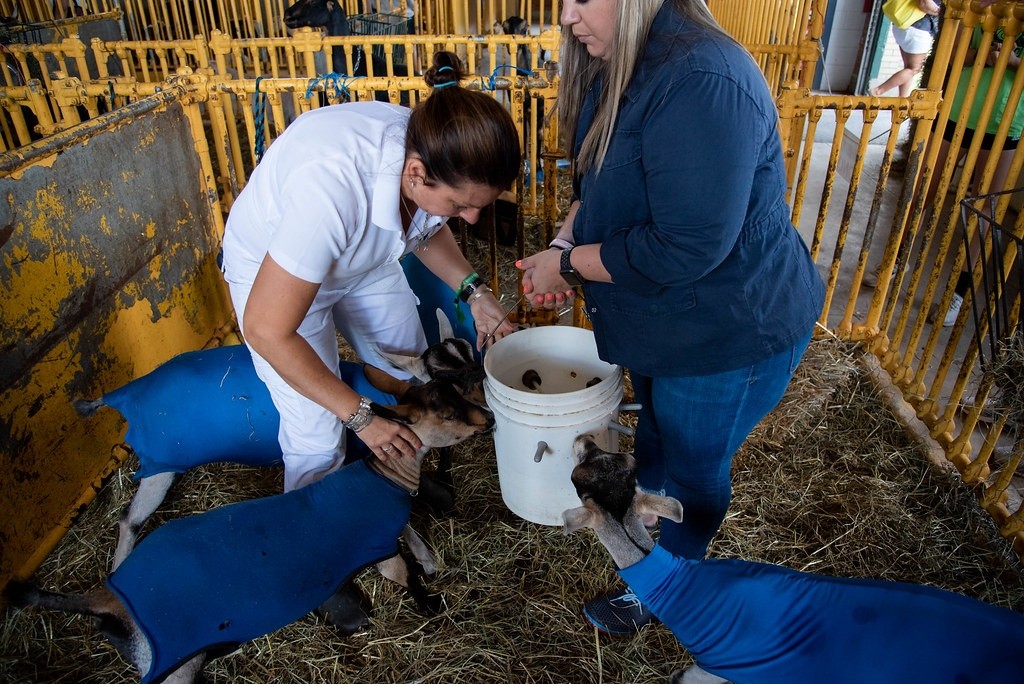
884;0;929;29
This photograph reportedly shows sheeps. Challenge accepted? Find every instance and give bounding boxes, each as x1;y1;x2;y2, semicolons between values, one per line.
560;431;1024;684
279;0;422;104
22;362;497;684
71;307;487;576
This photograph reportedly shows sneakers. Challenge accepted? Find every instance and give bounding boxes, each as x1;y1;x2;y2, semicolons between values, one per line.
585;586;651;635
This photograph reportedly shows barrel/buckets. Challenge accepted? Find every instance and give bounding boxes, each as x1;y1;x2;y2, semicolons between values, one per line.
480;292;624;527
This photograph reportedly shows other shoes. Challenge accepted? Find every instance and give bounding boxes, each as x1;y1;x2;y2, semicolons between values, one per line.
862;258;910;289
930;290;965;327
413;475;454;517
316;584;369;635
